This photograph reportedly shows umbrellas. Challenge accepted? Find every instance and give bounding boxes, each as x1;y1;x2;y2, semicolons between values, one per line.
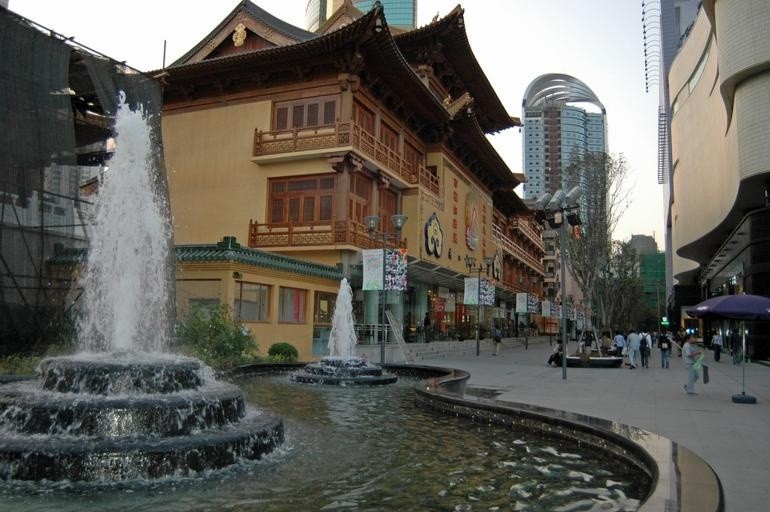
686;295;770;393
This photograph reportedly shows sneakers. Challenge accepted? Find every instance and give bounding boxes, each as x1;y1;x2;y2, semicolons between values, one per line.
683;384;688;391
688;392;698;396
630;365;635;369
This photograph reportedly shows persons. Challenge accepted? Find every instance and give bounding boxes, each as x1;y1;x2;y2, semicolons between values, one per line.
424;312;431;343
547;328;742;395
519;320;537;337
492;325;501;356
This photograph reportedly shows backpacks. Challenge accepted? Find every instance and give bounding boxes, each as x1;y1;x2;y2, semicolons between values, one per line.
661;339;669;350
605;337;611;349
640;333;649;347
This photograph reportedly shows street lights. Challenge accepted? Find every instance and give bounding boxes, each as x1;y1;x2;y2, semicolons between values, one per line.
518;276;539;350
534;184;585;377
542;286;558;345
463;255;494;356
364;213;409;365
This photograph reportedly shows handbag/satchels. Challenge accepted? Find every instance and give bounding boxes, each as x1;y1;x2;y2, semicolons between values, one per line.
495;336;501;343
621;347;627;356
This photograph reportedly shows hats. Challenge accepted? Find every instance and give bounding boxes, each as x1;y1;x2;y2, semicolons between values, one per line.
663;331;666;334
590;331;595;333
